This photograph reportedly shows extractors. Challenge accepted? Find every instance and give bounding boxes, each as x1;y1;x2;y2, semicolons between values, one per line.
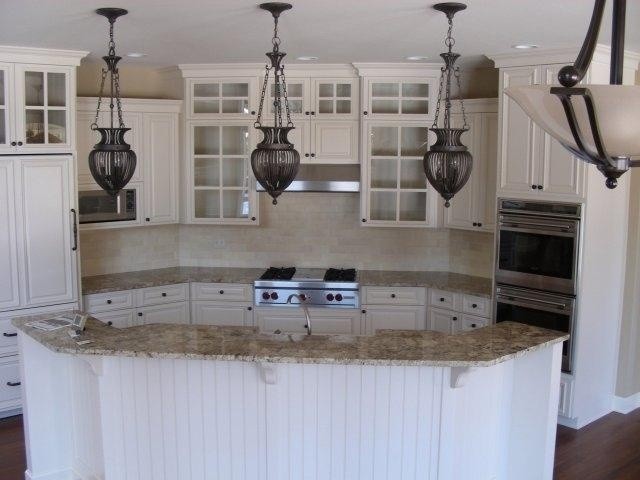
256;180;359;192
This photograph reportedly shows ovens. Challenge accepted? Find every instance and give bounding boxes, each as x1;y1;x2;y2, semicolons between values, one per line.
491;196;583;376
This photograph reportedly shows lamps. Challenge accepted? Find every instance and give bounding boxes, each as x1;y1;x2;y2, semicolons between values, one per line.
422;1;473;207
251;1;303;205
88;6;137;200
502;0;640;189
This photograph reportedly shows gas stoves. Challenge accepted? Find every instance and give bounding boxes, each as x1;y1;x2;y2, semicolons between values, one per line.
253;266;360;309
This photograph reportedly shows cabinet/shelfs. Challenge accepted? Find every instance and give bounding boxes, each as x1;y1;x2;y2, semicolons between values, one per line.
0;156;83;315
493;46;638;200
258;307;364;337
82;283;191;329
76;96;185;227
497;198;630;430
361;284;430;336
190;282;257;331
426;289;494;334
179;59;446;232
438;97;499;234
0;44;90;155
0;312;19;420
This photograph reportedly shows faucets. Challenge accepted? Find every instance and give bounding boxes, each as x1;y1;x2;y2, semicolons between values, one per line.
287;294;313;336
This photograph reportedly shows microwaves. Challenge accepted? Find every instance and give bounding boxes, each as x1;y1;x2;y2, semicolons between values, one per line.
72;189;139;223
